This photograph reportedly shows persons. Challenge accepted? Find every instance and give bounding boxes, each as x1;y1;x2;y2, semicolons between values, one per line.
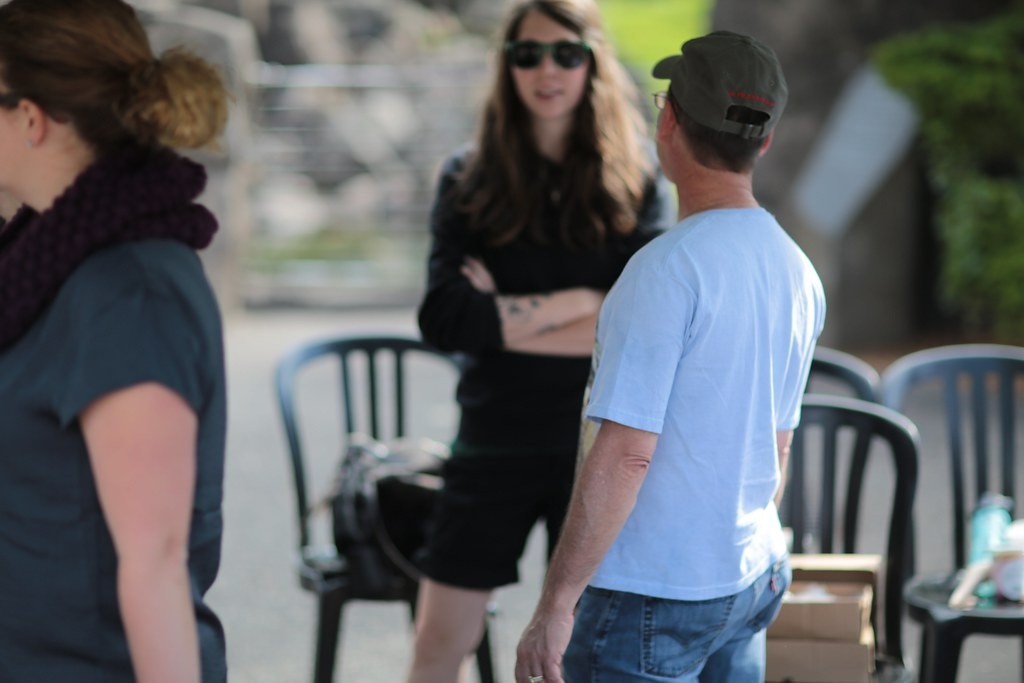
514;32;827;683
408;0;678;683
0;0;229;683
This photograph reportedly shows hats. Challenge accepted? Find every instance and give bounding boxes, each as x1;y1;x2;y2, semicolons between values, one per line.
653;30;787;139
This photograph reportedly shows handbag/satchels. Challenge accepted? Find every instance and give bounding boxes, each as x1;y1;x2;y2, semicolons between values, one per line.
334;433;455;567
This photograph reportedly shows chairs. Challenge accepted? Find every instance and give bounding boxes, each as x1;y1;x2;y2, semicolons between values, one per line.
274;333;496;683
880;344;1024;683
764;396;921;683
775;347;880;553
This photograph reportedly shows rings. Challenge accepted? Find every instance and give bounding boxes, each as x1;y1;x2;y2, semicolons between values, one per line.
528;675;544;683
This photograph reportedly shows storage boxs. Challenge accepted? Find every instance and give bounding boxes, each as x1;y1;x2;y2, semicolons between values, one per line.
765;629;872;683
766;582;871;641
790;554;878;598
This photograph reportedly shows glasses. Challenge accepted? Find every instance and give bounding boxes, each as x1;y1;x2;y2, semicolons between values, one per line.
505;39;588;69
652;90;671;108
